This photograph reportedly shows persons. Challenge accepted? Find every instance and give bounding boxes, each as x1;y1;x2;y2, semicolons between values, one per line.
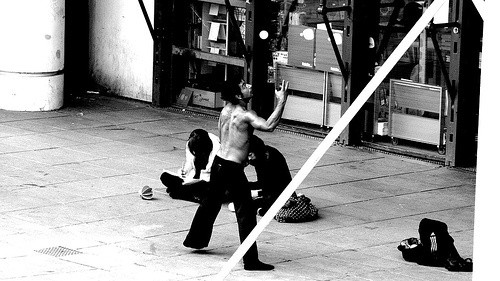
160;129;222;203
184;77;290;271
246;136;293;216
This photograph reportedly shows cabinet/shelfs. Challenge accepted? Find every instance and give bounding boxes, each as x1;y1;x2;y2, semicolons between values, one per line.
386;77;448;155
271;62;344;132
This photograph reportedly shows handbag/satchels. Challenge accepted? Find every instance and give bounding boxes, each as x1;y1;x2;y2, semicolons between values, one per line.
272;196;320;223
396;236;422;261
416;217;454;267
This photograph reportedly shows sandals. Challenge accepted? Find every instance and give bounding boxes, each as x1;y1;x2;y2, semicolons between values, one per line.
445;256;473;273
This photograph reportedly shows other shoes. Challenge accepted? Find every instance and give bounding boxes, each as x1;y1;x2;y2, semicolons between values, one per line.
228;203;236;211
243;259;275;272
